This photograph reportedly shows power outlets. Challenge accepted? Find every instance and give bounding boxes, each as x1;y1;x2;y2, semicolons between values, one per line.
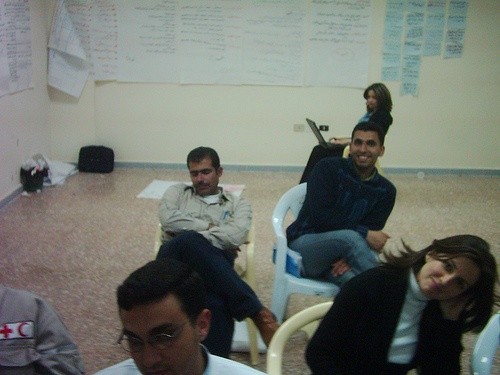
319;125;329;131
293;124;305;132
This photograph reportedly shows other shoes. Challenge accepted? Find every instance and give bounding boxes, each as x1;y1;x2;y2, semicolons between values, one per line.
258;306;280;346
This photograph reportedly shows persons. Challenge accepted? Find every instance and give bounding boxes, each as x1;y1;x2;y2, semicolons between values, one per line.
93;262;266;375
286;123;398;285
304;234;498;375
159;147;281;359
300;83;393;183
0;286;86;375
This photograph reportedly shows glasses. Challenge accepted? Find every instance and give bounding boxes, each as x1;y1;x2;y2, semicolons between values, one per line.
117;320;192;353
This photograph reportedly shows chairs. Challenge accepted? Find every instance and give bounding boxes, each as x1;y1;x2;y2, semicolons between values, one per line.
154;182;500;375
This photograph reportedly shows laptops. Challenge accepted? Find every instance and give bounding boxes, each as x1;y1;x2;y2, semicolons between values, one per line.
306;118;348;149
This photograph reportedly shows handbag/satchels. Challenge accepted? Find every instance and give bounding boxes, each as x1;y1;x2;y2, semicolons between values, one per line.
79;145;114;173
